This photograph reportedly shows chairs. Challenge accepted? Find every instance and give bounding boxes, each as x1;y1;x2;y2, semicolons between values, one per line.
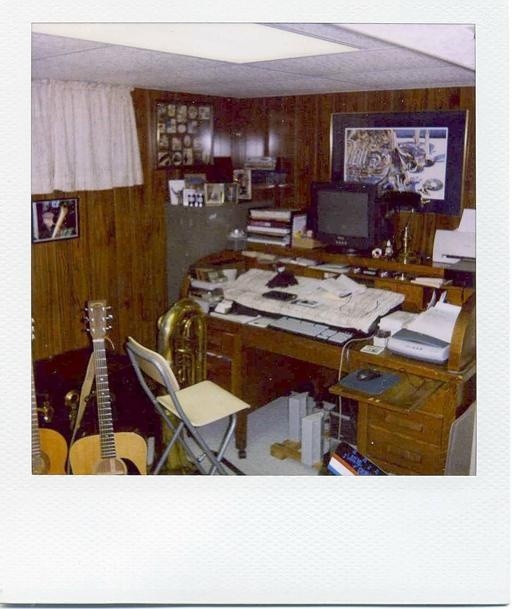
125;337;250;476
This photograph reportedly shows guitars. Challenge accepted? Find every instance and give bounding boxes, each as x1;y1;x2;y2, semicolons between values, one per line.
32;318;68;474
69;300;148;475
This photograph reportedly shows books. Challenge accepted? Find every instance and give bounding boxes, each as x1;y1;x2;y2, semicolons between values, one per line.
327;442;388;476
288;391;310;443
301;410;331;467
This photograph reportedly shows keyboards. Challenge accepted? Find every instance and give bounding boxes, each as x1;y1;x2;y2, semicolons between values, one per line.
268;315;357;347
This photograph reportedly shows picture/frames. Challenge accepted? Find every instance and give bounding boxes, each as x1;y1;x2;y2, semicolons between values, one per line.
31;197;82;245
328;109;469;217
204;168;253;207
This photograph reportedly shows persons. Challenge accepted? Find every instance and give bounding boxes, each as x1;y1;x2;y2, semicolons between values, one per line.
38;212;56;240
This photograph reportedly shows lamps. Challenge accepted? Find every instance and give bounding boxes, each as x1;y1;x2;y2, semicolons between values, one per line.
395;192;430;259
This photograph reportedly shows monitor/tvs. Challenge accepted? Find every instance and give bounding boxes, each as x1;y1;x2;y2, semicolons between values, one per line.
310;182;377;253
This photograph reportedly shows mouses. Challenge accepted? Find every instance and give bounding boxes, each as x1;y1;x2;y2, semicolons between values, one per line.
357;368;380;382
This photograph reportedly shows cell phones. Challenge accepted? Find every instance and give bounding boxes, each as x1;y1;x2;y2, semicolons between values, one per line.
263;290;296;301
297;298;320;307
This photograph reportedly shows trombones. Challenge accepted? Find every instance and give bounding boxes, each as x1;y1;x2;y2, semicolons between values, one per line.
155;296;207;474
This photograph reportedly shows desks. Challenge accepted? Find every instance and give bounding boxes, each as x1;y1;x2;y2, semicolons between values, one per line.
180;244;475;476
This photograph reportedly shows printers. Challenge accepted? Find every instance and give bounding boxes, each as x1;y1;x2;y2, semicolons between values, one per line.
387;328;451;365
